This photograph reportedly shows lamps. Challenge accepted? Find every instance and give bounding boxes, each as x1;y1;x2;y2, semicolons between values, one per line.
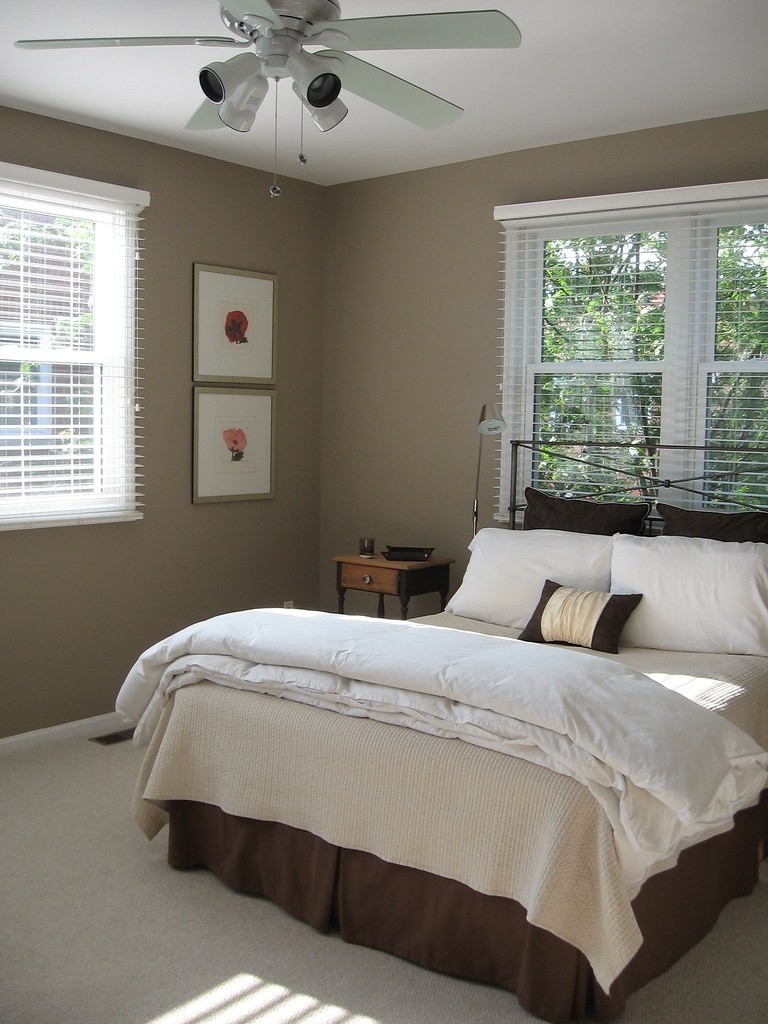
198;51;348;196
473;402;506;539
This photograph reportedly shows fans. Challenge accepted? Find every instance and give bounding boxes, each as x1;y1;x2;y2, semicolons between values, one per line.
14;0;522;130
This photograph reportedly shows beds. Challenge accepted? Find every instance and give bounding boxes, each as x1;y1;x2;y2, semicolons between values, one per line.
116;440;768;1024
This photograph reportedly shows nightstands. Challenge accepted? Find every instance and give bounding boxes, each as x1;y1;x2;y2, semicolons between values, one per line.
332;553;456;620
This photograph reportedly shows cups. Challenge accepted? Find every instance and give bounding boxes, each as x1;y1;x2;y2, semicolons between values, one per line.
359;538;374;559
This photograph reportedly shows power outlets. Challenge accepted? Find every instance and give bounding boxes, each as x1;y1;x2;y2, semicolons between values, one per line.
284;601;293;608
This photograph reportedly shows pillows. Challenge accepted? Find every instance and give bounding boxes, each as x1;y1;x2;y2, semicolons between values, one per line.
656;502;768;545
523;487;652;537
445;527;619;631
518;579;643;655
610;532;768;657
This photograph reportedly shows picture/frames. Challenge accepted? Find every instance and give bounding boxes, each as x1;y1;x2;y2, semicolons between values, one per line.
193;387;275;504
192;263;278;385
